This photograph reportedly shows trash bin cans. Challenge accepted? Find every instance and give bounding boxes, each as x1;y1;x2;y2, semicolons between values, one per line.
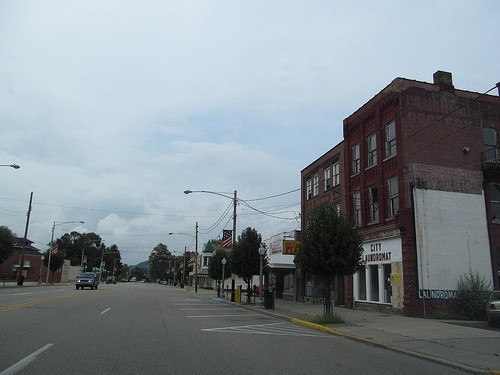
17;275;24;285
181;282;184;288
262;290;274;309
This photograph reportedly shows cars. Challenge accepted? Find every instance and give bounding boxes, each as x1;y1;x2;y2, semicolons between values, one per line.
75;272;100;290
105;276;118;284
158;280;168;285
121;277;137;282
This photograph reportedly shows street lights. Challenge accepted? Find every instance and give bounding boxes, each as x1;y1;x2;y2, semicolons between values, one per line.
46;220;85;284
220;257;227;299
258;246;265;305
168;221;199;293
184;190;237;302
